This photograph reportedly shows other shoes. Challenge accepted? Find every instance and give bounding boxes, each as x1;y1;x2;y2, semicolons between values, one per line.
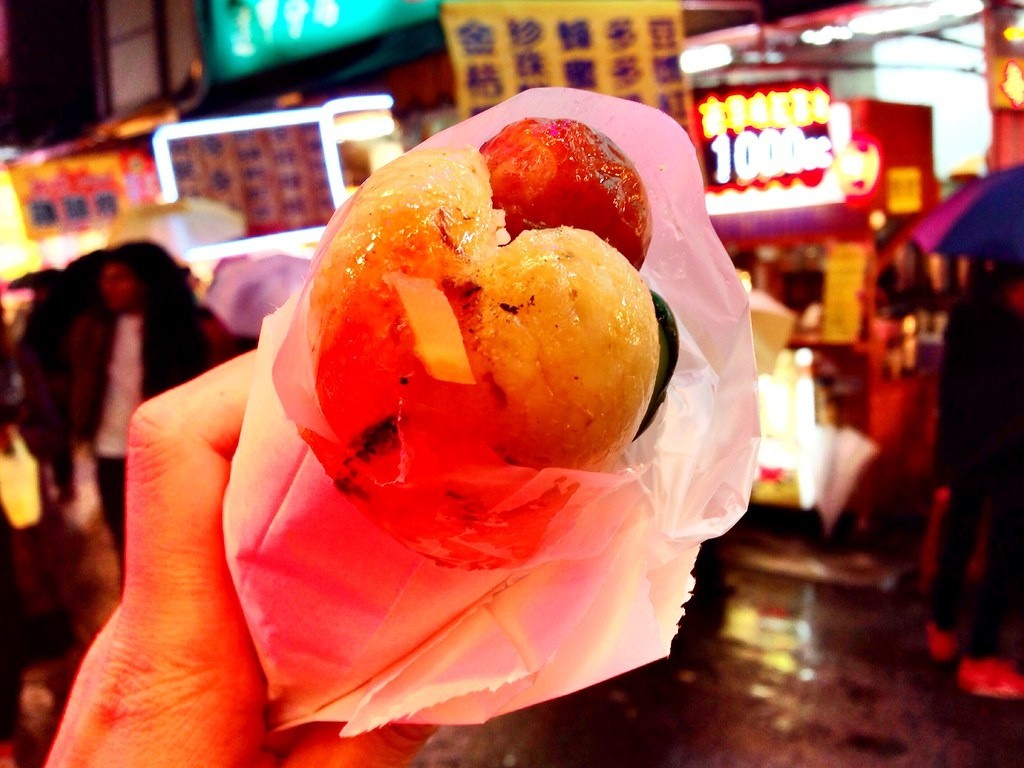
925;621;957;662
0;743;15;768
957;656;1024;700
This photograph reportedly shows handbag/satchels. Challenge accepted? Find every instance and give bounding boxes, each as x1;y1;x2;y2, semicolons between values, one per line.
0;430;42;528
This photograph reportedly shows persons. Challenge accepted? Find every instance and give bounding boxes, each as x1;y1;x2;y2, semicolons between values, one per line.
0;243;445;768
928;263;1024;700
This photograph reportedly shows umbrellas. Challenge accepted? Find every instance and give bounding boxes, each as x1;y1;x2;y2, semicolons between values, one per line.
109;194;324;334
912;165;1024;260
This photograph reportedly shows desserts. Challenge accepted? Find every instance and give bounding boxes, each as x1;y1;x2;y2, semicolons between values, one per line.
299;114;661;570
